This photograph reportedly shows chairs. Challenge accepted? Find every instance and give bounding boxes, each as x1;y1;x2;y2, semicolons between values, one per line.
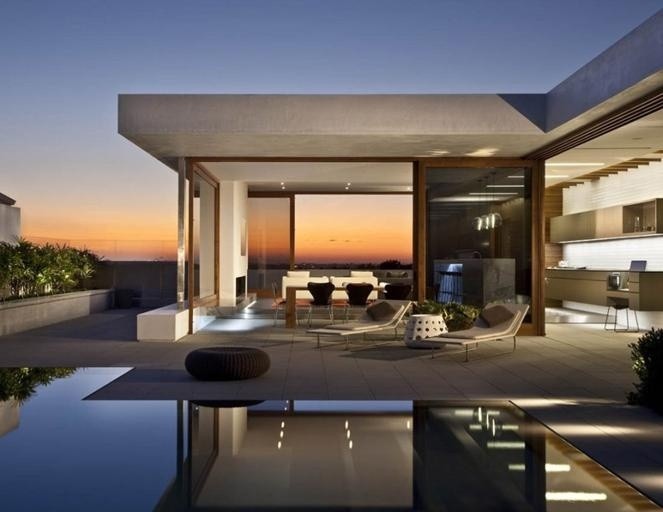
424;304;530;363
604;275;640;333
306;282;411;349
272;282;299;328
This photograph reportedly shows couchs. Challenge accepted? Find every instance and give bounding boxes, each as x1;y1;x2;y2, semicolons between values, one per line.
282;276;379;311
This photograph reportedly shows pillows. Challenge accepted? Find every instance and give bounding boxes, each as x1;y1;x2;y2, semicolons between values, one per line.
480;305;515;328
350;271;373;283
286;271;310;287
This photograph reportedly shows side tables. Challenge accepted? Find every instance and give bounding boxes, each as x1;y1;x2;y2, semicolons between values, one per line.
403;314;449;349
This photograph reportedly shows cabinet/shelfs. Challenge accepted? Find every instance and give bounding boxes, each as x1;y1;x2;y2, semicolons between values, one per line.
433;258;516;305
549;198;663;244
544;268;663;311
